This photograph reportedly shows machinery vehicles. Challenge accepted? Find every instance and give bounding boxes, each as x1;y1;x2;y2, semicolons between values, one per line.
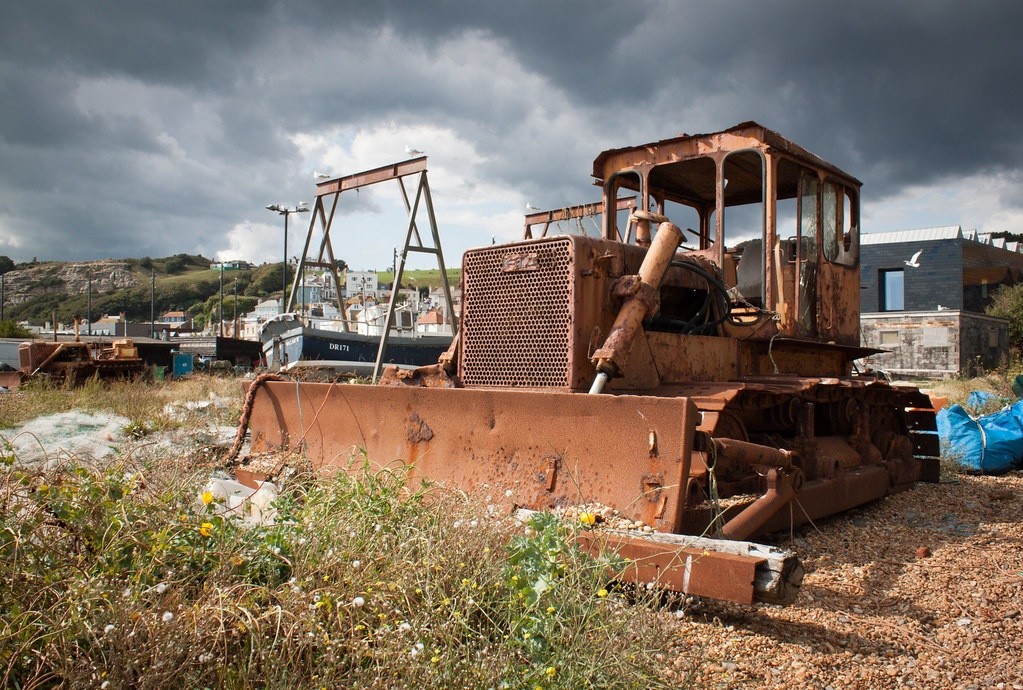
218;121;940;607
0;313;143;391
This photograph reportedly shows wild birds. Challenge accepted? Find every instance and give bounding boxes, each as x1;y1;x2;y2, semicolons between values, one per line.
904;250;922;268
313;170;330;183
936;305;950;311
405;145;424;159
526;202;539;213
299;201;307;207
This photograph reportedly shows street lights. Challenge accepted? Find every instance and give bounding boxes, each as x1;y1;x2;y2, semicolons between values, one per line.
265;204;310;316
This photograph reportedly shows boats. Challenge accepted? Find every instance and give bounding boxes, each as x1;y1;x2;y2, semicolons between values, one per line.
257;313;453;371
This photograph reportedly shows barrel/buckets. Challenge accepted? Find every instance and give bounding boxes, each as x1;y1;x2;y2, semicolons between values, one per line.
152;365;165;379
966;390;988;413
1012;375;1023;397
